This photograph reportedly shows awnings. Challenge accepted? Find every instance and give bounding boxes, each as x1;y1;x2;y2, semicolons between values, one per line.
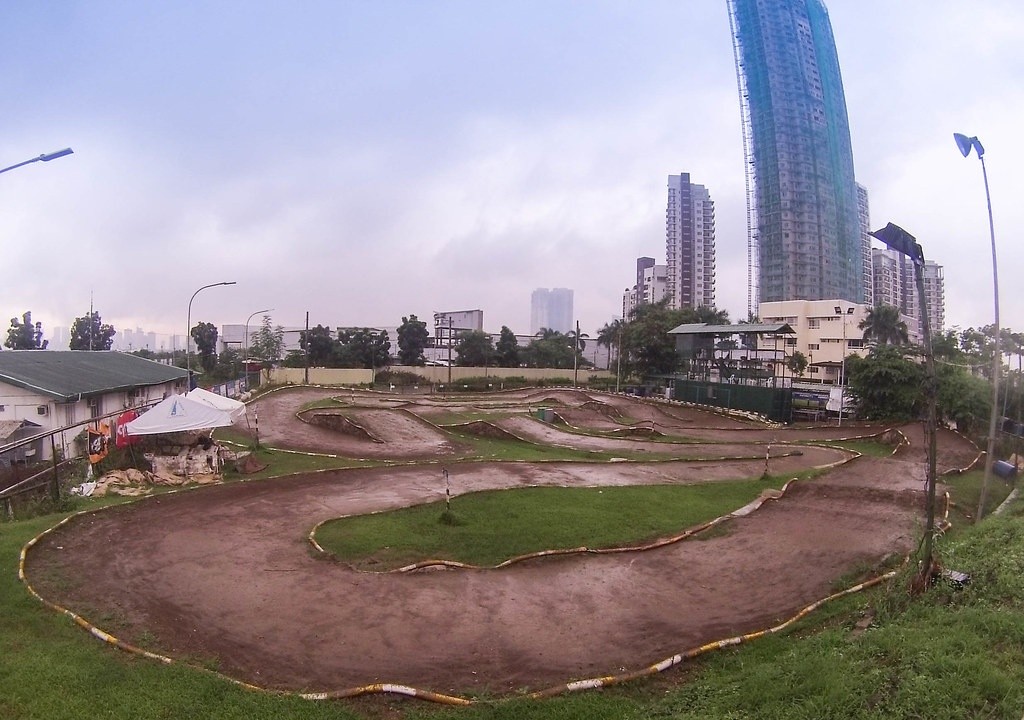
809;359;845;368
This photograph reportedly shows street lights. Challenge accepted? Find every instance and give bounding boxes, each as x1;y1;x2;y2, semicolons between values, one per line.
484;336;490;377
245;309;269;389
866;222;938;579
568;345;577;388
834;307;854;426
952;129;1001;524
187;281;236;392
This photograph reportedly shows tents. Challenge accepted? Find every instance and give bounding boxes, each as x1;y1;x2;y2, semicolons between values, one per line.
124;387;257;484
